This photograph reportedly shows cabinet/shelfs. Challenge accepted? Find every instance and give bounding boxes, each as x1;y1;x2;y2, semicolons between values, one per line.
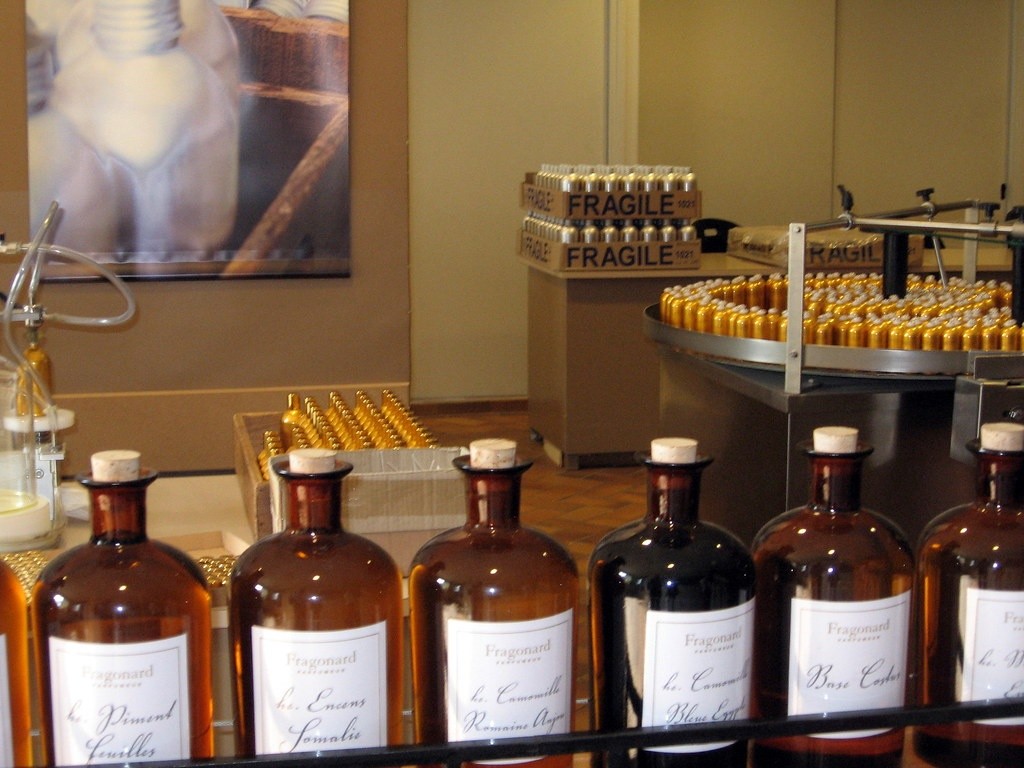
232;410;286;543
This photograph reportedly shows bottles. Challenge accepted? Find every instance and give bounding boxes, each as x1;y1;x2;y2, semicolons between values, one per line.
587;437;757;768
751;425;915;768
15;344;50;417
915;423;1024;768
522;210;696;244
228;448;404;757
0;449;215;768
659;272;1024;351
25;432;60;521
257;389;442;481
408;438;578;768
535;165;697;192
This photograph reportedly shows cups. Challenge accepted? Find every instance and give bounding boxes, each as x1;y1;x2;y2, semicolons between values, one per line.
0;372;38;512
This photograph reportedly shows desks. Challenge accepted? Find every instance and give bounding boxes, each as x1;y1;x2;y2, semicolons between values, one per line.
524;247;1014;470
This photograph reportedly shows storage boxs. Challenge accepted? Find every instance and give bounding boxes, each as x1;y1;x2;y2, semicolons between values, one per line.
515;171;704;272
728;224;927;267
268;445;471;578
148;530;252;756
